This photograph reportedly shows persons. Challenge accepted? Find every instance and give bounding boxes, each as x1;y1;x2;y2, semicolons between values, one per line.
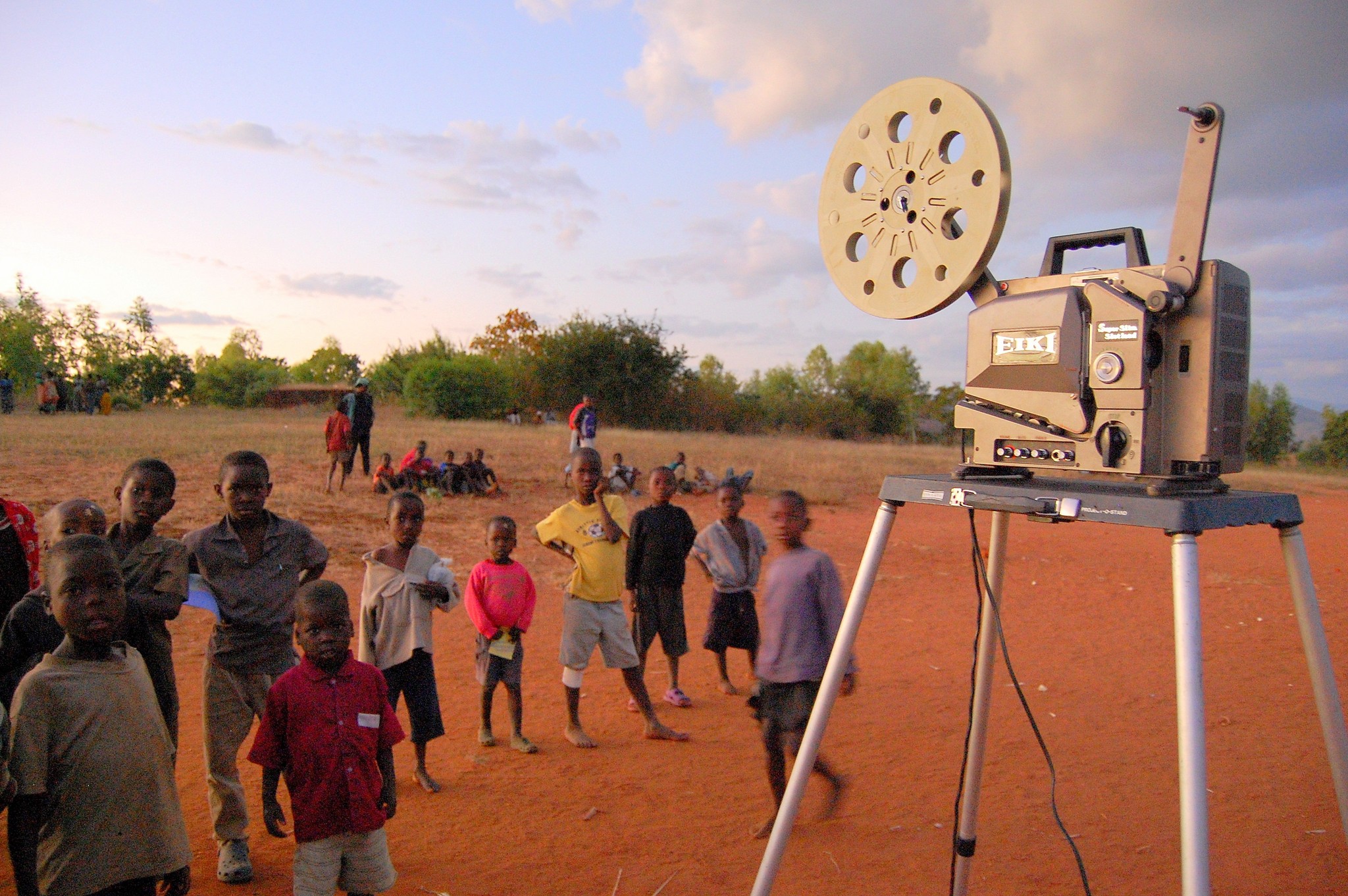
341;378;374;477
181;451;331;881
463;516;538;753
749;490;859;839
373;440;508;499
0;370;111;415
0;457;190;896
690;481;768;694
324;401;354;493
624;465;696;713
358;491;461;793
247;579;404;896
511;394;755;496
532;447;688;748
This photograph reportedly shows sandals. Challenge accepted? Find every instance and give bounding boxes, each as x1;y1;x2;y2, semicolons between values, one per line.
628;698;640;711
664;689;692;706
216;842;252;882
